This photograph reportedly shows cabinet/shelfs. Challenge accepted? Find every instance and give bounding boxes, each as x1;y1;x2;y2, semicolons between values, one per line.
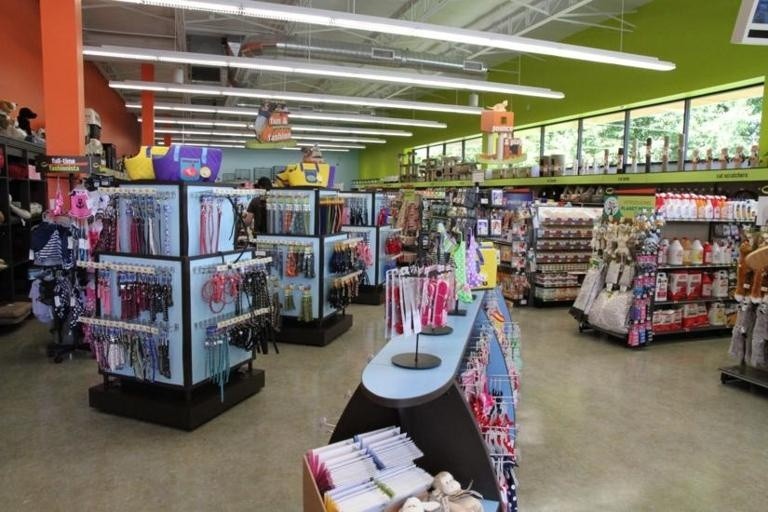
568;189;760;351
485;185;605;310
715;244;768;398
88;178;266;432
328;287;523;511
0;133;47;330
339;189;407;307
252;182;366;348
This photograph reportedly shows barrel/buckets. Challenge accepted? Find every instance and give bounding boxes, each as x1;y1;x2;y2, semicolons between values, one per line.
702;272;712;297
680;236;693;265
668;238;683;266
661;237;670;264
692;238;704;265
712;270;729;298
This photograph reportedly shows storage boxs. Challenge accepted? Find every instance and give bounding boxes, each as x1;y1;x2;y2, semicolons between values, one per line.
302;448;431;511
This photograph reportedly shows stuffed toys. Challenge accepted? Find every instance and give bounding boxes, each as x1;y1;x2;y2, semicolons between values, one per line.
0;98;19;129
14;106;38;136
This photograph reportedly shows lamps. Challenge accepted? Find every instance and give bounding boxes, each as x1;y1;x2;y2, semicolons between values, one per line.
154;129;387;144
82;0;568;99
124;101;448;129
154;137;367;149
157;141;350;153
108;72;486;116
137;117;413;138
117;1;677;72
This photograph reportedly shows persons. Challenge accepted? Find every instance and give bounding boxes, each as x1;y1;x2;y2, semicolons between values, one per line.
242;176;272;239
